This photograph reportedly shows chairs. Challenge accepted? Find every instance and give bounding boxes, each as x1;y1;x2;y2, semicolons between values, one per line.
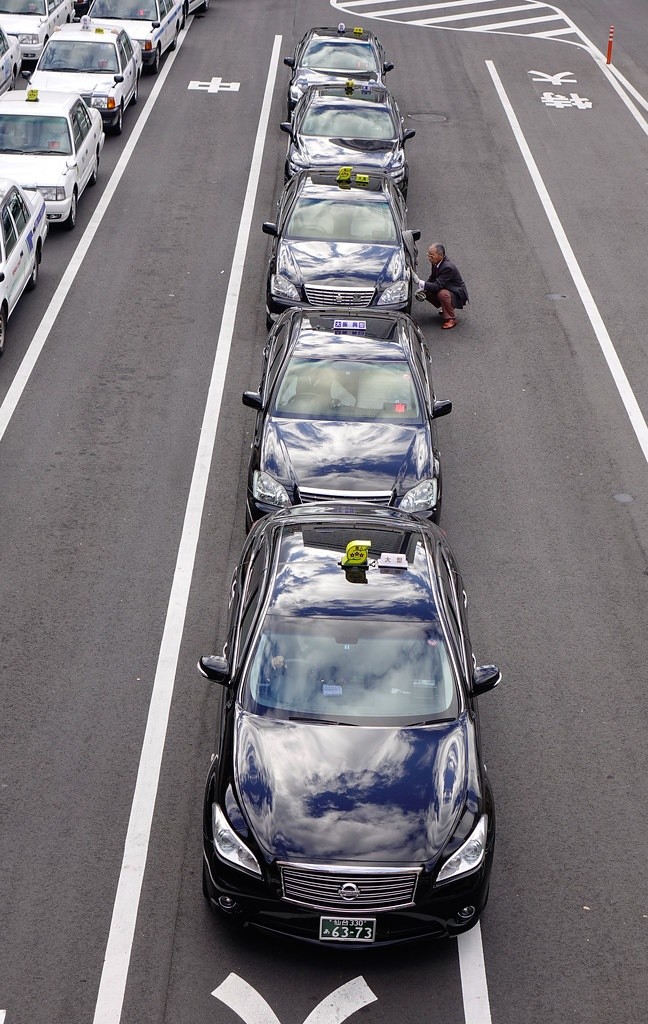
38;120;70;152
92;47;116;70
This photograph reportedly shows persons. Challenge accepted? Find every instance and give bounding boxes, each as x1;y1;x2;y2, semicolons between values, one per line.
267;637;334;703
411;243;471;329
20;0;43;14
279;366;356;408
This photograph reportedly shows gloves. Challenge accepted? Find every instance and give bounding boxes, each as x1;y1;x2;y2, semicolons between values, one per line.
411;268;419;284
415;288;423;296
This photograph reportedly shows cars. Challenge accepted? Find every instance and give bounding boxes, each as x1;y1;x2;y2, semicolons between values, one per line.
260;164;423;333
284;23;396;122
0;0;75;61
0;24;22;96
21;15;144;136
0;88;105;231
243;306;452;537
279;78;416;203
198;498;502;952
73;0;210;74
0;175;48;357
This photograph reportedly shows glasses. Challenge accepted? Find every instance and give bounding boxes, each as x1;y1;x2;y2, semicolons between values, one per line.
425;253;437;258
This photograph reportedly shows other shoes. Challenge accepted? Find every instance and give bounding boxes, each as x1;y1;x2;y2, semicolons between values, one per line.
442;320;456;328
